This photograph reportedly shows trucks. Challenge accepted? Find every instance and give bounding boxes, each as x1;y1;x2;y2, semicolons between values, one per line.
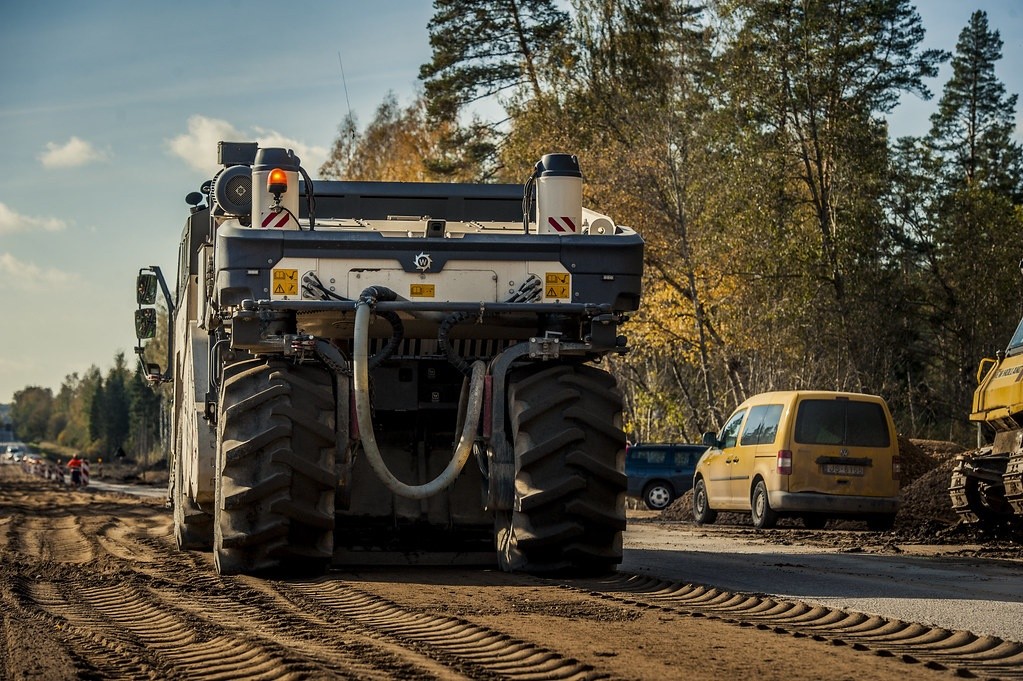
134;140;647;581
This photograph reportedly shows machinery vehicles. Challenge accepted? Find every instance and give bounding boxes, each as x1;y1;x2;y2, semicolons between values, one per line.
947;318;1023;537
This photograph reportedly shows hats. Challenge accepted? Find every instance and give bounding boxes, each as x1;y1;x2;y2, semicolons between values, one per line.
73;453;78;458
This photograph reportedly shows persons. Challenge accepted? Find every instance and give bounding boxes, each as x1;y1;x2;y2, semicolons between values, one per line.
39;454;82;487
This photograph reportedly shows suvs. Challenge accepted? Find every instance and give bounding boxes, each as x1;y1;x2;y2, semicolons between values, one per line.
627;443;710;510
693;391;899;531
